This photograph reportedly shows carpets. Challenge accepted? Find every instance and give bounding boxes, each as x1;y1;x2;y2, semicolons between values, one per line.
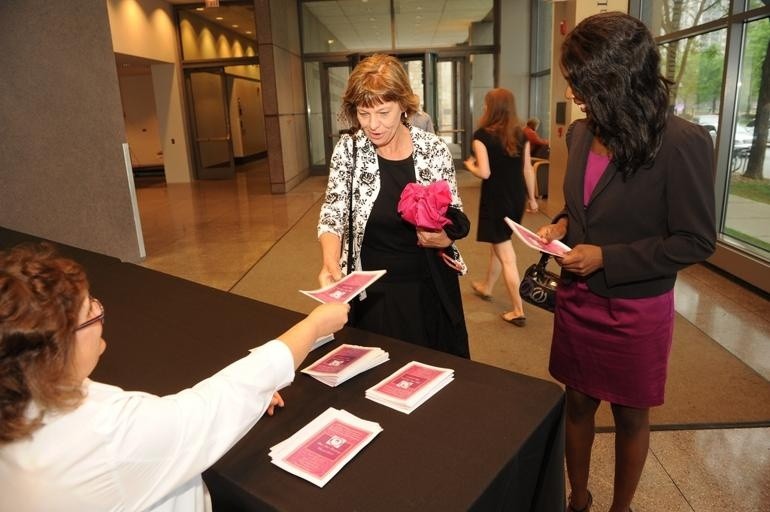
230;186;770;432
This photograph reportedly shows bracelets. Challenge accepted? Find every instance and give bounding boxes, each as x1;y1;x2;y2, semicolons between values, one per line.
528;199;536;204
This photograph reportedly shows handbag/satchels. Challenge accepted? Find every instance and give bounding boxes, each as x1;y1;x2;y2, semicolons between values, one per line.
518;252;561;315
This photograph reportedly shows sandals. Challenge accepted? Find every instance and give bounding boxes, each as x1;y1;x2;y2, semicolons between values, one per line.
471;281;492;301
498;311;527;328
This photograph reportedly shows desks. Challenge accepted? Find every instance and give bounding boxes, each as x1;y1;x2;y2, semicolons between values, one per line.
0;226;566;511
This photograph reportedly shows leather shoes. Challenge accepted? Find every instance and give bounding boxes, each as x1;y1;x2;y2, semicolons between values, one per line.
564;488;592;512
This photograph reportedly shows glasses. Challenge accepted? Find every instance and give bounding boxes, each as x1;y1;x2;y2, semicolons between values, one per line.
73;295;105;331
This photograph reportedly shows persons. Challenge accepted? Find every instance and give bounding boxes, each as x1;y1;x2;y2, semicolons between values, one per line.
535;10;718;512
0;239;351;512
522;118;550;156
463;87;540;327
316;53;470;361
405;93;436;135
